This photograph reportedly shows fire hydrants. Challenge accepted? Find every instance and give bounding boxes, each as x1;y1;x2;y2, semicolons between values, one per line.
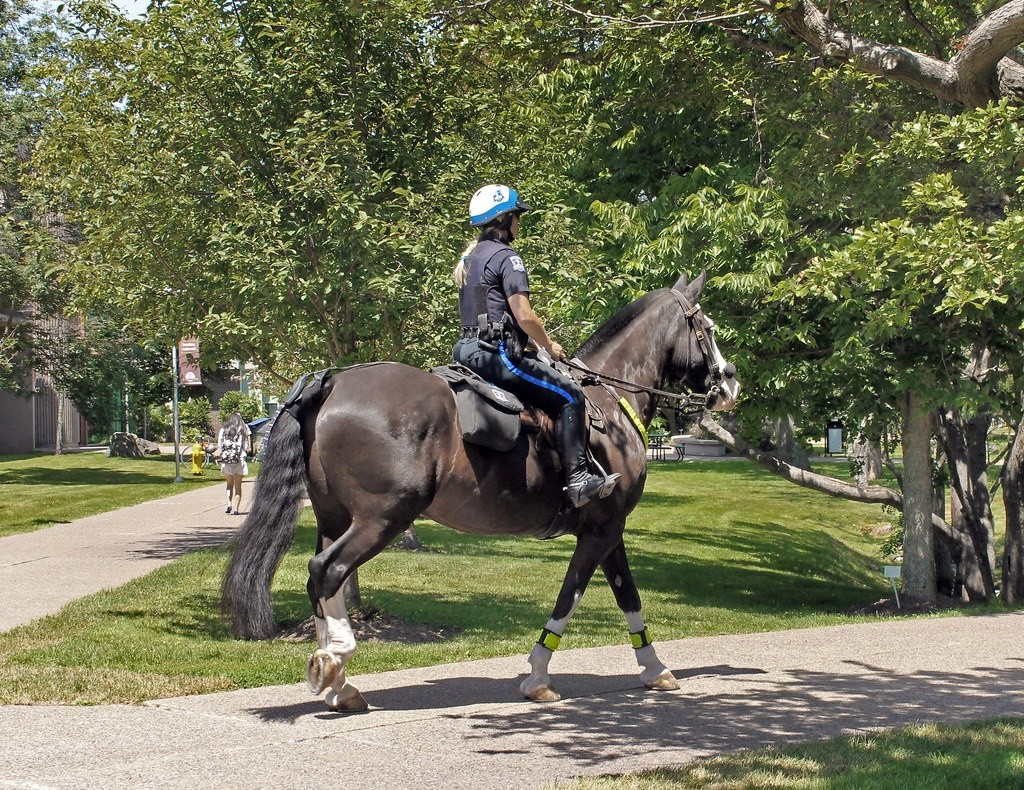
190;441;203;477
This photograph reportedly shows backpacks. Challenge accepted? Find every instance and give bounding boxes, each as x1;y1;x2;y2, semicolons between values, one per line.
220;429;244;464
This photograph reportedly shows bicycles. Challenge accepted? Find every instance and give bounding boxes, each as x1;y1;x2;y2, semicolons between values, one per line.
181;432;211;471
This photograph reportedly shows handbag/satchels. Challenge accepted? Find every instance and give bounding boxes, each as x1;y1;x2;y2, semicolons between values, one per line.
447;363;524;453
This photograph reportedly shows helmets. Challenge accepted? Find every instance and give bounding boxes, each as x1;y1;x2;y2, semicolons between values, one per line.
469;184;533;228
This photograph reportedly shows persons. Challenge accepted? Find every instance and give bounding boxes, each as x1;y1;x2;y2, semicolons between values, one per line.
217;412;252;516
450;183;624;510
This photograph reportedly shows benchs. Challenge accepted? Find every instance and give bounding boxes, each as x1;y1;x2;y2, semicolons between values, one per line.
671;444;685;461
648;444;661;460
647;447;672;462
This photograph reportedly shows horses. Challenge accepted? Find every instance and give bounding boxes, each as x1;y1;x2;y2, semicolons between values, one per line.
218;268;743;713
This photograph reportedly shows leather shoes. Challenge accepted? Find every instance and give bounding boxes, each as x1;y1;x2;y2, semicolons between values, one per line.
566;472;622;508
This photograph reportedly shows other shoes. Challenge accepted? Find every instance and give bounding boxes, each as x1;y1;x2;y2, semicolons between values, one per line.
225;506;238;515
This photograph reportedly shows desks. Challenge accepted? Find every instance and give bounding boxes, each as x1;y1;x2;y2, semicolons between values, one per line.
647;433;669;461
